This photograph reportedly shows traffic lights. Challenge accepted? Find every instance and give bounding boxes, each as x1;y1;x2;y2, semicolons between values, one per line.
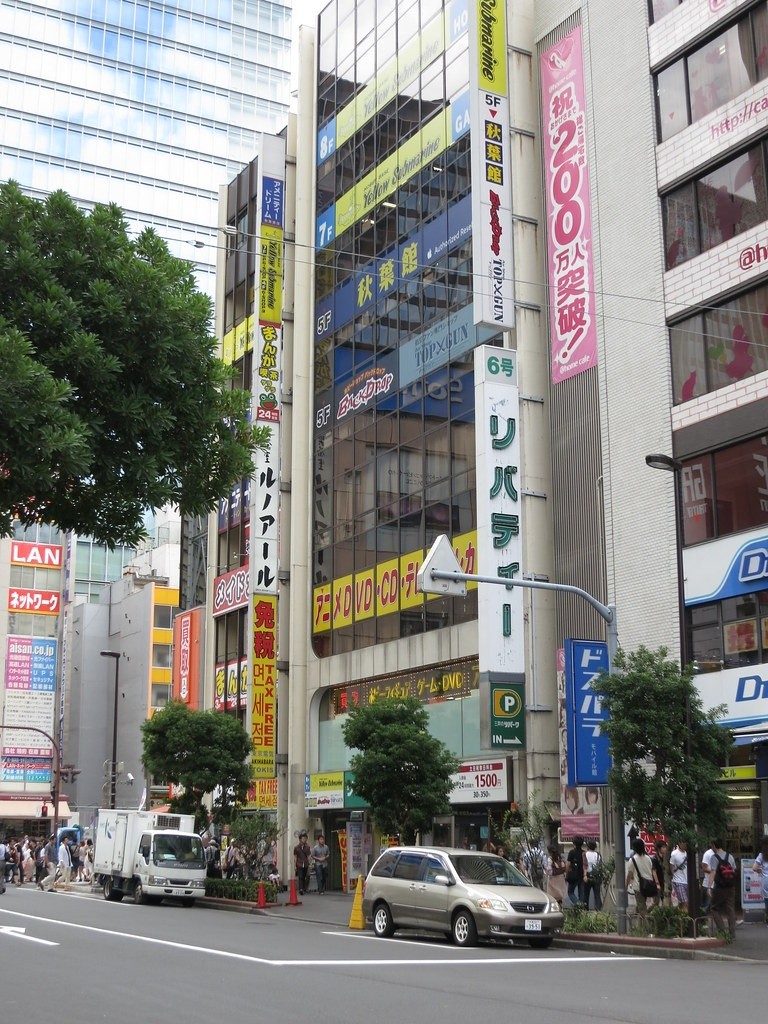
51;791;55;805
54;768;68;782
41;806;48;817
71;769;81;783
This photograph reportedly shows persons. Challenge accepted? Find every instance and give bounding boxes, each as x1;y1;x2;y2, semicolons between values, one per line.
495;838;566;905
647;835;688;912
311;834;330;895
583;786;600;814
201;835;244;880
0;834;95;894
563;836;603;910
699;838;737;941
752;835;768;927
562;784;584;815
627;839;654;915
293;834;311;895
260;835;274;880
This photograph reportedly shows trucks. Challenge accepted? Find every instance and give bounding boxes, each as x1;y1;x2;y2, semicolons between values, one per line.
49;827;92;862
92;808;213;906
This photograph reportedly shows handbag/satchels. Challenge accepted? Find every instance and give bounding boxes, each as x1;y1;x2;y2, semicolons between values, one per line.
586;869;604;883
223;861;230;872
566;869;578;882
4;852;10;861
639;877;657;896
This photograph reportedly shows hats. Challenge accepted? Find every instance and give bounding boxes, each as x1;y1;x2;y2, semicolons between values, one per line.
231;838;236;843
208;839;216;845
587;839;596;850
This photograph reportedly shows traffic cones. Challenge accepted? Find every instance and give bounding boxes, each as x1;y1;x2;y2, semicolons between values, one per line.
285;880;303;906
252;881;271;909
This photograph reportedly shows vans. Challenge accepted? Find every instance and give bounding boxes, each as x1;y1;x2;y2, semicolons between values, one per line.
361;846;565;948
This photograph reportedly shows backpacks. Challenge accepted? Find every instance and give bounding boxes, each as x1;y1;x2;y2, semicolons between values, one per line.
530;850;543;881
552;857;566;875
713;851;737;887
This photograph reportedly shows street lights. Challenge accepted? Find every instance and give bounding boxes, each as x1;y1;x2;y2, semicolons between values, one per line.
99;651;121;807
644;451;710;938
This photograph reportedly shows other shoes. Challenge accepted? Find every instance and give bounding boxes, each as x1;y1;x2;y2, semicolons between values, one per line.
735;920;743;927
78;878;82;882
299;890;303;895
702;924;708;929
48;888;56;892
38;883;44;891
84;874;86;880
